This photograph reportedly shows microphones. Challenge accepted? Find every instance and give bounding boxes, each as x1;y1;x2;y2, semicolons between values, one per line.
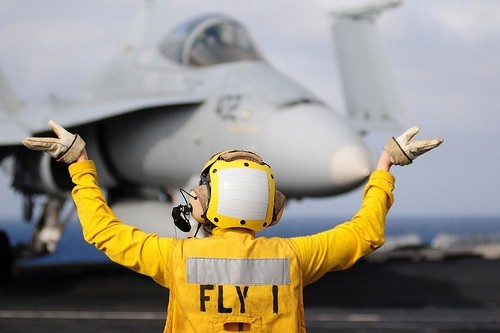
172;206;193;232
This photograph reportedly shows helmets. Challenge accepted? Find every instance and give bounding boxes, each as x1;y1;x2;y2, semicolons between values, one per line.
199;148;277;232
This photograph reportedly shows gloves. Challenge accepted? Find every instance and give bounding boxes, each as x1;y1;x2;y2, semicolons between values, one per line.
20;120;85;164
384;127;445;167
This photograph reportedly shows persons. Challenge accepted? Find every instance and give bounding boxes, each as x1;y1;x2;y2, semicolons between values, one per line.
22;121;443;333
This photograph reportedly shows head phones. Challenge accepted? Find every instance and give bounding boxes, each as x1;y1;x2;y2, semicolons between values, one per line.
189;150;287;226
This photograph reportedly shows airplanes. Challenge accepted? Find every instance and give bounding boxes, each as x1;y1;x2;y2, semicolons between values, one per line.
0;8;373;293
